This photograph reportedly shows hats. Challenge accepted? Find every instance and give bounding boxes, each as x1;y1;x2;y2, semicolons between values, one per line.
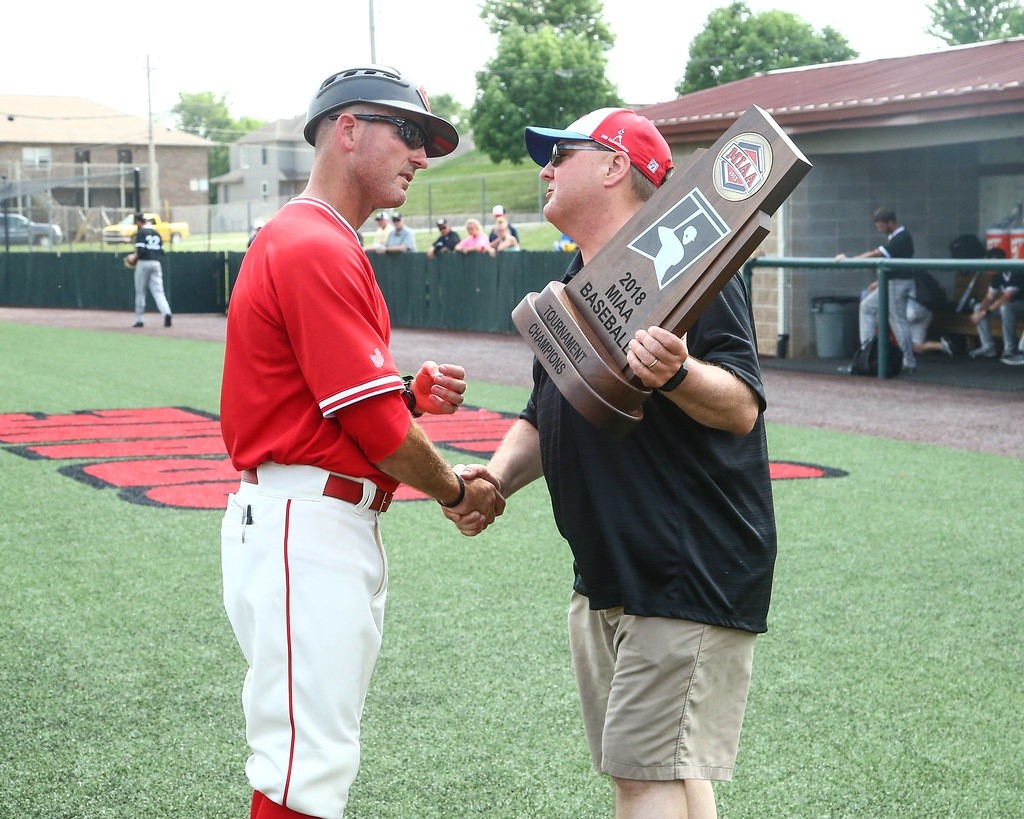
392;211;403;222
374;212;388;220
133;212;145;224
493;206;505;216
523;106;674;187
253;220;265;231
436;218;447;227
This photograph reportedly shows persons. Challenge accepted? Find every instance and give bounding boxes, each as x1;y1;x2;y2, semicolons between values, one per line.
447;106;780;819
216;62;509;819
248;203;521;256
123;210;177;328
835;203;959;376
968;247;1024;367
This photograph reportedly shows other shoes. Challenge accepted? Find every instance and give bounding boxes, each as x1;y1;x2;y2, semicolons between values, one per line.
940;336;956;357
898;366;916;376
968;345;1024;366
133;322;144;327
165;314;172;326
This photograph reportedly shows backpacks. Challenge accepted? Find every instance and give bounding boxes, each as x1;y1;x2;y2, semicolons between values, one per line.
851;336;902;379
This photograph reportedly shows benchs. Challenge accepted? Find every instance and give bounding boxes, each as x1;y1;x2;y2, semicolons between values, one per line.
935;270;1024;338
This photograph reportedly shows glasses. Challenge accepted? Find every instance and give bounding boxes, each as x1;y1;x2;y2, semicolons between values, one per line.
328;114;433;154
546;142;612;166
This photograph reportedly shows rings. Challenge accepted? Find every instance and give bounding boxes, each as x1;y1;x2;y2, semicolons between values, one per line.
646;358;660;369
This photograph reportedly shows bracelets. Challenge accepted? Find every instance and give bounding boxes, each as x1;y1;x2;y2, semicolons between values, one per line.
985;307;993;316
401;375;424;419
653;364;689;394
435;471;466;511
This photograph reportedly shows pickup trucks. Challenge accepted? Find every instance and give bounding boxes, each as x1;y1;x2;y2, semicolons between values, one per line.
102;213;189;244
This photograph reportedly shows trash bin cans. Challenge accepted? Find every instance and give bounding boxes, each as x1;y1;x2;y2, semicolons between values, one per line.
811;295;860;360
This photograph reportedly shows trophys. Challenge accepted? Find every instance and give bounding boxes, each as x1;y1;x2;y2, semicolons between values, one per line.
511;100;813;436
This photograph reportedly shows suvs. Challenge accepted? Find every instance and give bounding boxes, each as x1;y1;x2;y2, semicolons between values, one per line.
0;213;63;246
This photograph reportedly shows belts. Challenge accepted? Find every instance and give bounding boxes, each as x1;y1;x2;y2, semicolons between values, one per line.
241;467;395;516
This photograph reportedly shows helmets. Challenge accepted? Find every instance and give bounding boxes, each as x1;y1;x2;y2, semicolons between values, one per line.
303;64;459;158
